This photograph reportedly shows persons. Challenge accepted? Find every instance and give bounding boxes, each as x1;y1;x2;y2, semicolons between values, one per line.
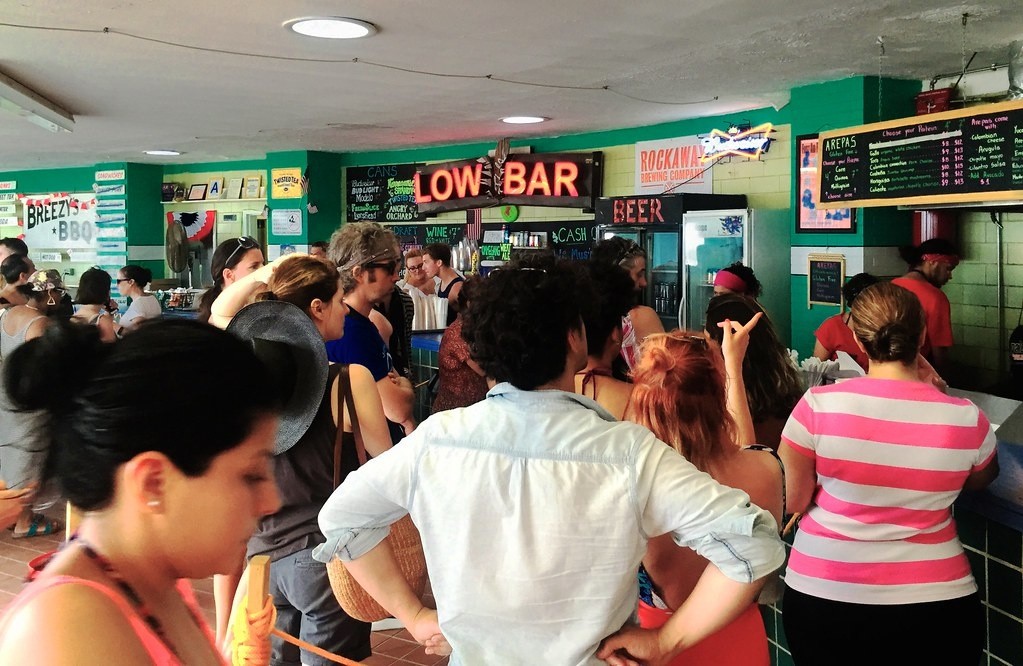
626;311;785;666
0;238;162;539
777;282;999;666
574;237;965;450
0;315;282;666
312;266;786;666
197;221;497;666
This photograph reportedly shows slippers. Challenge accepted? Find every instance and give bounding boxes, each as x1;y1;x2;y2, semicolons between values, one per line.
5;512;64;538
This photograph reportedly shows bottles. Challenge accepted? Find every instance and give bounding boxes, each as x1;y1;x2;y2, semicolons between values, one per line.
411;235;418;244
654;283;676;316
148;282;151;291
513;231;541;247
707;268;720;284
502;224;513;244
154;286;186;310
450;236;480;276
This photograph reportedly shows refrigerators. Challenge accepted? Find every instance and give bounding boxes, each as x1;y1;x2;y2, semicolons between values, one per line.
594;195;755;339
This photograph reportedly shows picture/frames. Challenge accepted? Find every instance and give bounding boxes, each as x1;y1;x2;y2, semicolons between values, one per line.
186;184;208;200
244;175;262;198
205;176;224;199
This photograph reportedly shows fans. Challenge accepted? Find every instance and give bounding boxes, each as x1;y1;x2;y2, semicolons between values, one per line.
166;219;201;289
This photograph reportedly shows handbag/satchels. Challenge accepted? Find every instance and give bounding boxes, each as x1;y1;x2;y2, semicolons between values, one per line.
325;513;426;624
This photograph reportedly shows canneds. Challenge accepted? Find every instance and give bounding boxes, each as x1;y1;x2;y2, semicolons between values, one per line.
509;231;542;247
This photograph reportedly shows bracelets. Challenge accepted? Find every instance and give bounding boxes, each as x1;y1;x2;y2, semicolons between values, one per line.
117;326;124;335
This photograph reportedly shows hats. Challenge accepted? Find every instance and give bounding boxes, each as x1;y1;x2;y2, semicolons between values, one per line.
226;300;329;457
27;269;71;291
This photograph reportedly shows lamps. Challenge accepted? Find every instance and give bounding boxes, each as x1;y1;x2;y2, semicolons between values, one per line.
0;73;76;135
283;16;379;39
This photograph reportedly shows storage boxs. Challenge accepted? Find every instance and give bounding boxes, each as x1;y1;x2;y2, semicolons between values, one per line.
151;278;180;291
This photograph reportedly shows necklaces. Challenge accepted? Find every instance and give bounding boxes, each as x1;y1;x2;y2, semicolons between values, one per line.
25;304;39;312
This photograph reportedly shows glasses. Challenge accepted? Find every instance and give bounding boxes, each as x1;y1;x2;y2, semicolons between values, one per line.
406;264;423;274
224;236;261;267
117;279;130;284
363;258;401;276
619;238;637;267
677;335;708;350
487;267;549;290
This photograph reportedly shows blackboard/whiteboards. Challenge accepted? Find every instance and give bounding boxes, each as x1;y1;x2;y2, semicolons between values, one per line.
347;163;429;221
380;221;599;279
807;256;845;307
818;107;1023;210
